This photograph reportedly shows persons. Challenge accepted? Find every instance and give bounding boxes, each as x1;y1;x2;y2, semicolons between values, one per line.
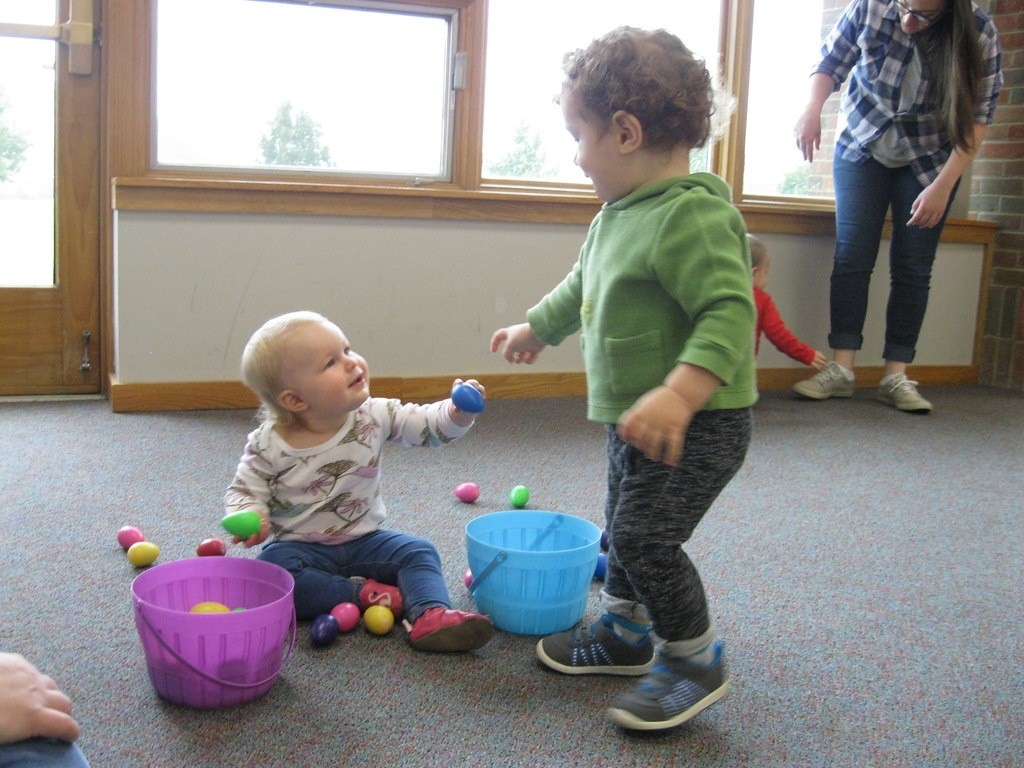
791;0;1004;415
488;25;759;732
745;233;829;371
0;652;89;768
223;311;494;653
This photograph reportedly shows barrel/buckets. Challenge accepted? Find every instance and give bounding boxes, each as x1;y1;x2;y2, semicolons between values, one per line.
464;509;603;635
131;555;296;710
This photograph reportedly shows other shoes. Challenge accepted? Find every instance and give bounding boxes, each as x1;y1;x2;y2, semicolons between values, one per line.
409;605;493;652
359;579;404;622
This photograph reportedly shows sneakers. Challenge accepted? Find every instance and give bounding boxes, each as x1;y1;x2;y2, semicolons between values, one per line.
794;361;858;398
537;614;655;674
606;639;730;730
874;373;932;410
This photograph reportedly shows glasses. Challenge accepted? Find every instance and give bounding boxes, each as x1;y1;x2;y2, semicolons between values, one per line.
894;0;953;26
